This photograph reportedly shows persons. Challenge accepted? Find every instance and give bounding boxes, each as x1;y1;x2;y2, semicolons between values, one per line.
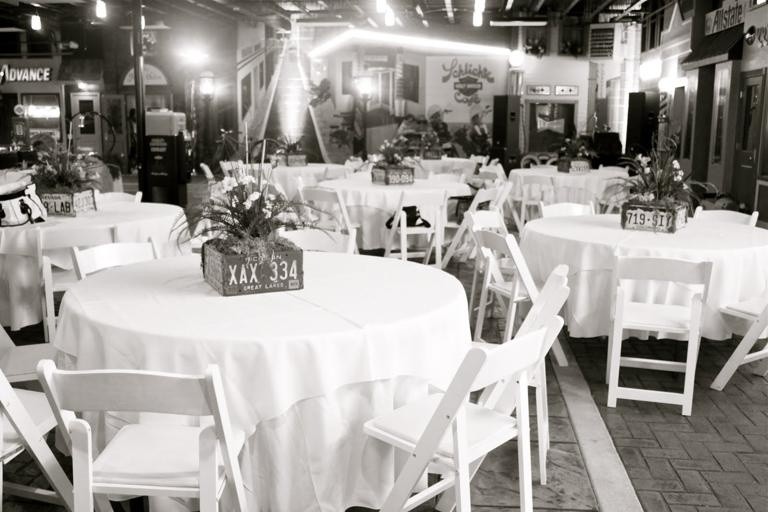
428;105;453;157
465;114;489;159
397;115;420;147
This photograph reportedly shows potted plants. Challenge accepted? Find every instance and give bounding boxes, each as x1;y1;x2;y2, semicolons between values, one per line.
371;135;415;187
606;132;720;233
170;122;343;297
28;136;105;218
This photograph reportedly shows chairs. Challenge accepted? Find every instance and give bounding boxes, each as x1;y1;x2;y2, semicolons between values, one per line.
469;153;490;176
33;226;118;344
381;188;446;272
37;358;248;512
537;198;595;221
463;210;523;330
463;263;571;487
0;321;53;393
71;237;160;282
470;225;569;368
603;254;714;417
218;157;246;179
709;297;768;392
199;162;218;194
0;365;73;512
294;186;361;255
441;186;501;276
361;316;566;512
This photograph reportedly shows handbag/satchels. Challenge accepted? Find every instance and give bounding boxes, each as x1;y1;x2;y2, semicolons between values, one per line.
0;181;49;229
